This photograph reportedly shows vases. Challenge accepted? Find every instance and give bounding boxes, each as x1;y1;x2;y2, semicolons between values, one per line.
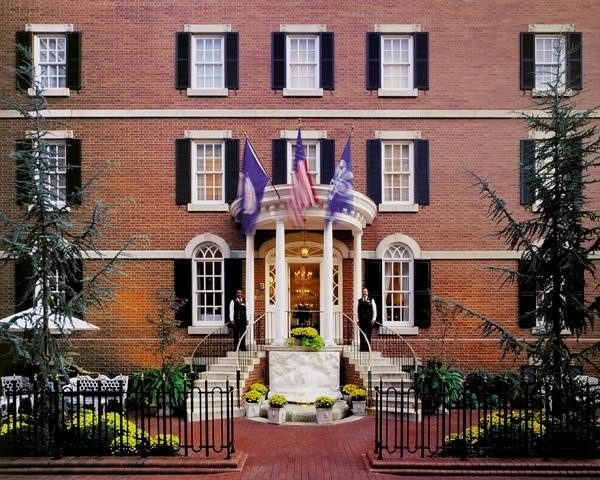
259;394;267;406
268;407;286;425
293;338;302;346
316;407;333;424
244;401;259;417
343;393;350;401
352;400;366;416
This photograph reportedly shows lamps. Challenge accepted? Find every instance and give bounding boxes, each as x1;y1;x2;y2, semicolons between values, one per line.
293;286;316;298
293;265;314;286
299;218;311;258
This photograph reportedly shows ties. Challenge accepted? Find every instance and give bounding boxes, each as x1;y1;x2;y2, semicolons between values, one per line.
364;297;366;301
239;299;241;304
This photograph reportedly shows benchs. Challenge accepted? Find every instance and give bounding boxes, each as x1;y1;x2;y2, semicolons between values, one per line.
0;371;129;418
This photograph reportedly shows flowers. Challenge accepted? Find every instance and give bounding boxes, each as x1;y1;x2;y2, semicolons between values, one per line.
314;395;336;408
342;383;360;395
249;383;268;395
268;393;288;408
241;390;263;402
347;388;367;401
290;327;318;338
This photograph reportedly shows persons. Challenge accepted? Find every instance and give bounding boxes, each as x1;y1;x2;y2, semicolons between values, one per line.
354;287;378;353
229;288;251;352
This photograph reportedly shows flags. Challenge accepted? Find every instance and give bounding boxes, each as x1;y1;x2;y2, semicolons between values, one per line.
285;116;319;231
234;130;272;240
322;122;357;232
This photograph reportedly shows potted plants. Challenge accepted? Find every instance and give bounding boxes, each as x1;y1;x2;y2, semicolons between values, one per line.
412;356;465;415
130;361;192;417
